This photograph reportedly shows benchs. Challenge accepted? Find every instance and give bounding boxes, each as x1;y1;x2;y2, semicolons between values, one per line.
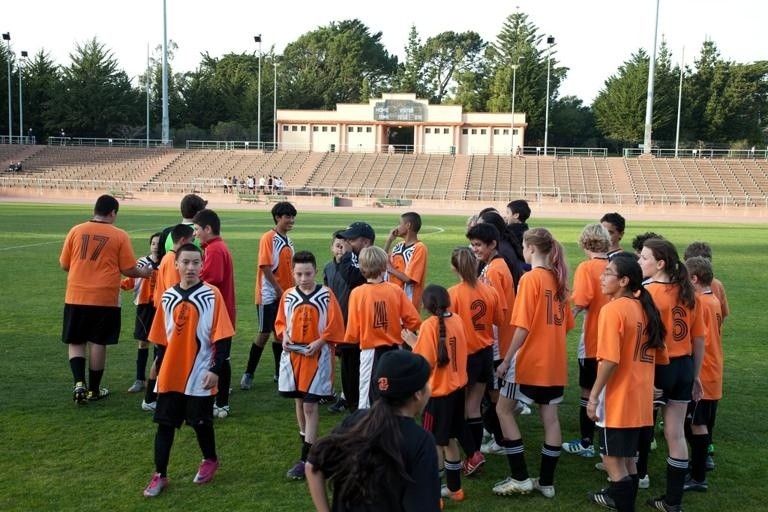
1;142;763;209
108;186;134;200
375;198;401;207
236;194;259;204
266;195;297;206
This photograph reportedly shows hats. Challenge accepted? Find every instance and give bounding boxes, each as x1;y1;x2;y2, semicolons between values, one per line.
371;350;431;394
335;221;374;240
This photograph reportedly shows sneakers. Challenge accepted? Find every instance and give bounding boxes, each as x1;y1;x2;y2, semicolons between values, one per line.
441;483;464;502
591;487;617;511
683;474;708;491
193;458;219;484
647;494;682;512
480;437;506;456
144;473;168;497
492;477;555;498
561;439;595;458
128;379;145;393
213;403;230;418
240;372;253;390
461;451;486;477
320;392;349;413
73;382;109;404
287;460;305;480
142;398;157;410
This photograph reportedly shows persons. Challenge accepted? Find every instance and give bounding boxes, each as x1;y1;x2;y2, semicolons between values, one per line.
14;161;22;172
569;212;730;511
515;145;521;155
303;349;442;511
60;127;67;146
750;145;755;157
60;195;152;405
9;161;16;172
223;174;284;195
240;200;574;498
120;194;237;418
142;243;236;496
28;127;33;143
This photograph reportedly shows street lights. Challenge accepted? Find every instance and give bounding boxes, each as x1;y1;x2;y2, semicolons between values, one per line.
272;63;281;151
141;37;151;149
674;42;688;156
254;33;262;152
543;34;555;156
2;31;13;144
510;65;520;156
18;51;28;145
